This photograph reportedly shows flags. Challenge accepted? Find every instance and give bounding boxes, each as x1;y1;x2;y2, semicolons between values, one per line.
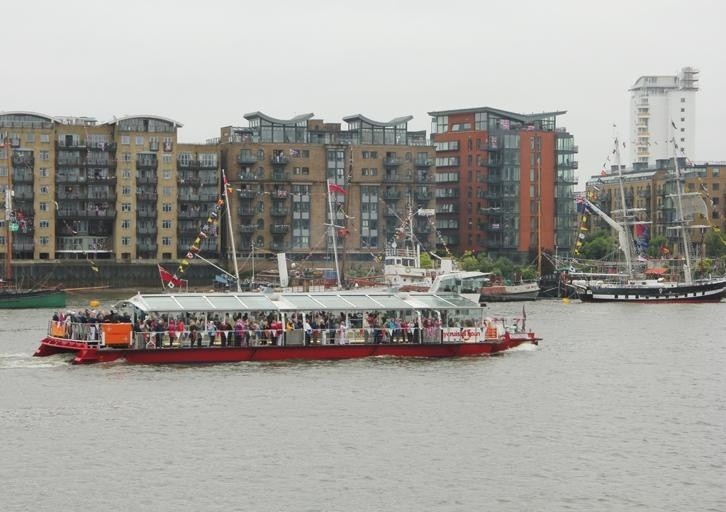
166;184;234;289
327;179;348;196
158;261;186;287
555;135;725;275
221;168;231;187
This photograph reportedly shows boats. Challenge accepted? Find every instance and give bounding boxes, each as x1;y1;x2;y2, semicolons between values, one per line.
213;167;540;301
34;289;544;367
555;123;725;303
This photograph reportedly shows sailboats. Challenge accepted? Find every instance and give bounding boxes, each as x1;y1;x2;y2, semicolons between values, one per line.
1;135;68;309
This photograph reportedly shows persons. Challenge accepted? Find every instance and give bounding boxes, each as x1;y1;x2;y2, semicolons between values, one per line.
50;303;483;349
